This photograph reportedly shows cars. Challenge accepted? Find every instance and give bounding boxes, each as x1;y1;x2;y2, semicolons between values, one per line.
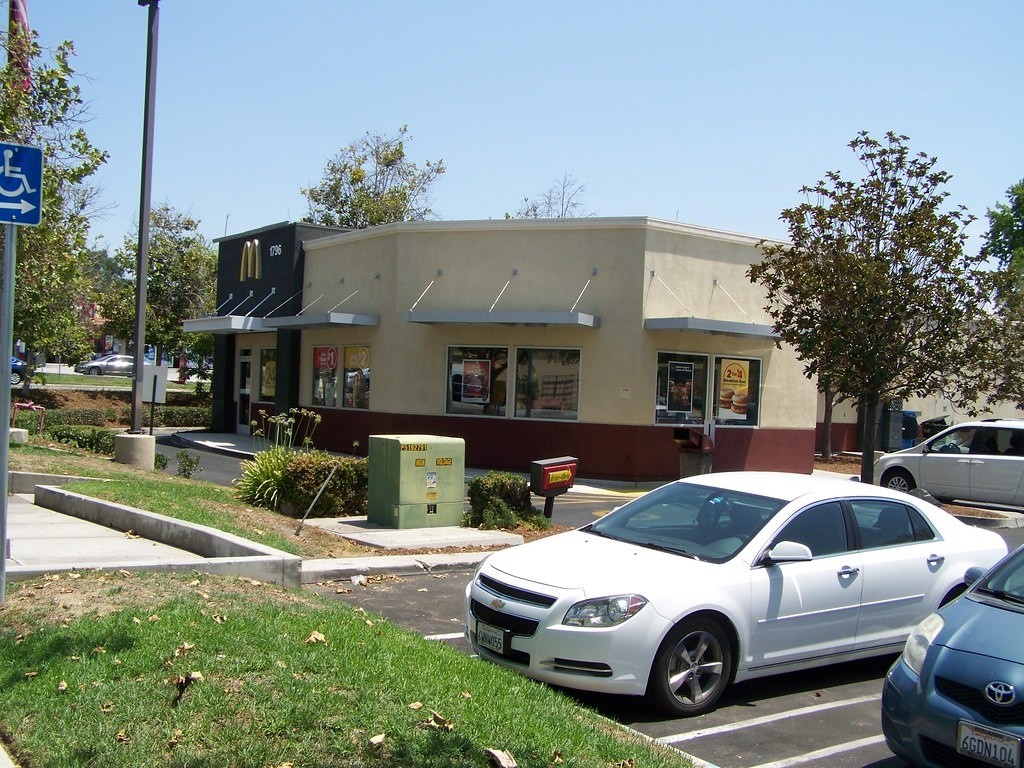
463;470;1009;718
880;530;1024;768
75;354;151;378
12;354;34;385
872;416;1024;510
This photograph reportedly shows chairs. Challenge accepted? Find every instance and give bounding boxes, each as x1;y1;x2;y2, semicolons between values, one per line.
1004;435;1013;454
708;504;762;542
860;505;908;546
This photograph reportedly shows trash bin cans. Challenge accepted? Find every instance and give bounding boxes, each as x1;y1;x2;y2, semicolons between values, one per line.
903;410;922;448
920;414;955;446
674;426;716;480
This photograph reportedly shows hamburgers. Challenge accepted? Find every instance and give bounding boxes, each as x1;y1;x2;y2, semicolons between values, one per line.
719;389;747;414
